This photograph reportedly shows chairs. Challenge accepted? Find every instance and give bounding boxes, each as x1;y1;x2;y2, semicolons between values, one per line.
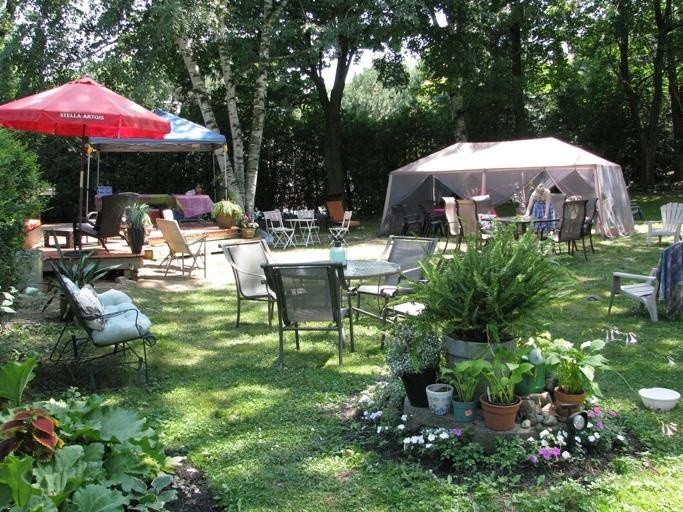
260;261;357;371
158;209;204;269
648;201;683;242
391;193;598;261
379;251;481;353
155;218;208;280
607;242;683;323
346;235;439;331
75;195;130;252
219;238;309;333
261;200;362;245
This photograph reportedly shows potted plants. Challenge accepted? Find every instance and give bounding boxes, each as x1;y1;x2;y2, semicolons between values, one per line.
399;330;610;431
384;217;581;404
124;204;148;253
44;250;123;322
211;201;258;238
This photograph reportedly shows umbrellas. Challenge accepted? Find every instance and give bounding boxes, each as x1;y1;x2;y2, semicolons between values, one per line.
0;76;172;249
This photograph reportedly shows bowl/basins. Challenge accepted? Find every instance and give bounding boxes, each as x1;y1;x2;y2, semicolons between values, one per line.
639;387;680;411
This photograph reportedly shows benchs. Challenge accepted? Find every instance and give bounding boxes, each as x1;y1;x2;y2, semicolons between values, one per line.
47;259;158;382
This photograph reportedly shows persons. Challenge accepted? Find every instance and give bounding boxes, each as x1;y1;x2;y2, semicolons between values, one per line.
524;183;555;235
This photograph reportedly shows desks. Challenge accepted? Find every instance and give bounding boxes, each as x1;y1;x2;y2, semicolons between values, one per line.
305;259;401;350
95;191;213;225
45;226;90;250
